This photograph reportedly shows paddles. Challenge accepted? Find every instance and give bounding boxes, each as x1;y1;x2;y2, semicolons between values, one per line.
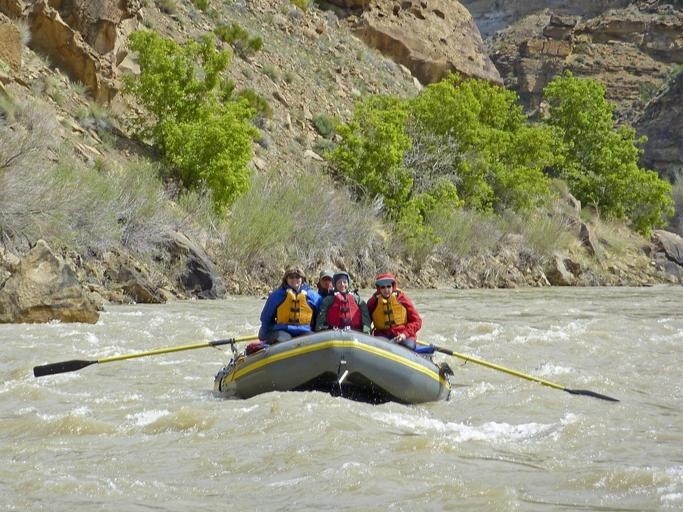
415;340;618;401
34;335;259;377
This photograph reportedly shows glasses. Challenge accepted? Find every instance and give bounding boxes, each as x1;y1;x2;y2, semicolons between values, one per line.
379;285;393;290
286;275;304;280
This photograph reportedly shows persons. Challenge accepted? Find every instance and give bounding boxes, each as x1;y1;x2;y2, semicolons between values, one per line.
256;267;325;345
312;269;335;298
365;271;435;358
314;270;371;337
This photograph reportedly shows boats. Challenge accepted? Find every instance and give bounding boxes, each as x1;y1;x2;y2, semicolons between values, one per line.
214;328;453;401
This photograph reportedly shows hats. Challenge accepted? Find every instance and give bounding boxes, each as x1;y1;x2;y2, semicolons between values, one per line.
283;267;305;279
319;269;334;279
